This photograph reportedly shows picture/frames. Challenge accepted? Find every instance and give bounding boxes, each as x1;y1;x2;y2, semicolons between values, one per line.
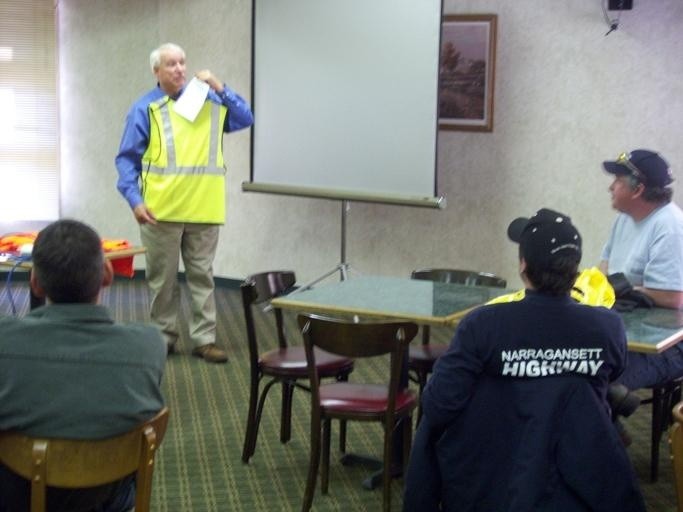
434;11;501;135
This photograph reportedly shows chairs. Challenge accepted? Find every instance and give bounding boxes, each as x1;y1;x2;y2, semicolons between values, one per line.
395;263;509;433
233;268;357;468
0;403;172;512
606;370;681;492
289;302;430;512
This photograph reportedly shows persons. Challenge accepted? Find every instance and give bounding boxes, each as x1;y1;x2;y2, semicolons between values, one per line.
402;208;649;512
599;149;682;448
1;218;169;511
114;41;255;363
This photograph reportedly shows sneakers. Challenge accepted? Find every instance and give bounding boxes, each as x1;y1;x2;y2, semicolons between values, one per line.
192;345;227;361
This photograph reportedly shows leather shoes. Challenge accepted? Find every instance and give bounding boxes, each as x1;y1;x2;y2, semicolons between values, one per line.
609;384;640;418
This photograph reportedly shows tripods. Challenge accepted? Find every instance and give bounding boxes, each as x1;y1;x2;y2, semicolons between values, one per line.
262;204;361;324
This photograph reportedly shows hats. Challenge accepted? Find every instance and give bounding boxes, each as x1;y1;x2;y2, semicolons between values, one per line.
604;149;674;187
508;209;582;272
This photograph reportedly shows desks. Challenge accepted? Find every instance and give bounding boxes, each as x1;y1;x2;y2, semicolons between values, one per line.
268;269;682;495
0;241;152;313
